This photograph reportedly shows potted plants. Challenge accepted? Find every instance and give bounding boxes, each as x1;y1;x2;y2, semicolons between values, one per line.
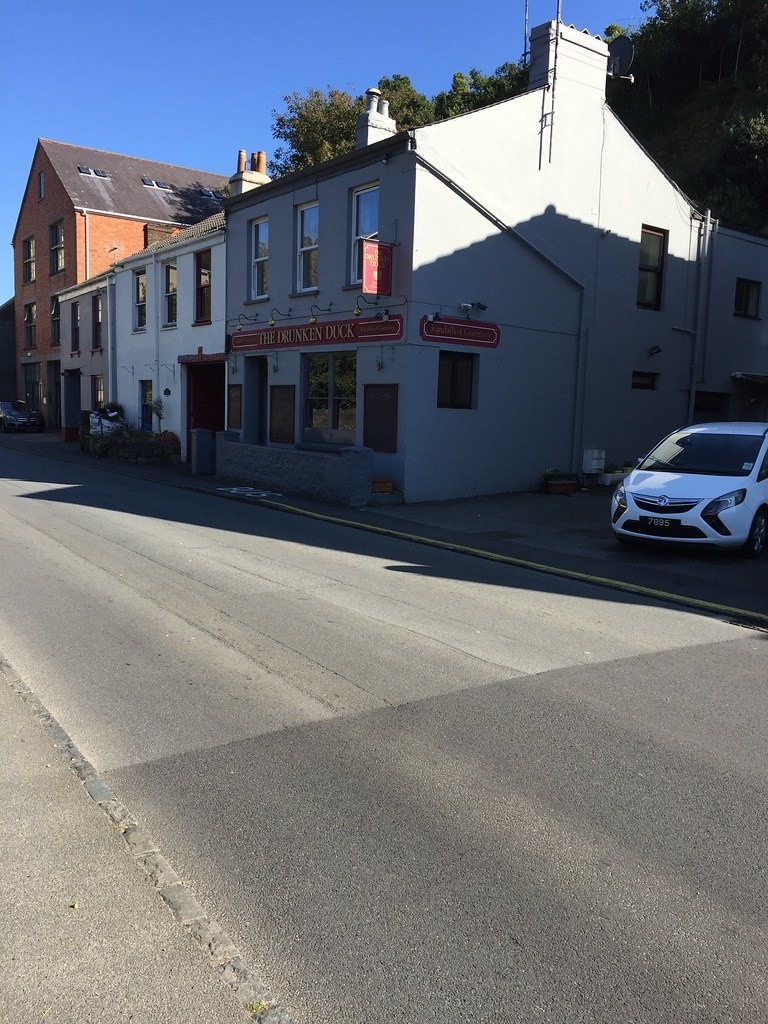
542;467;577;493
609;461;634;473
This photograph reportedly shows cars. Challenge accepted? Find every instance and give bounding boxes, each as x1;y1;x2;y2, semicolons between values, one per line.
611;423;768;559
0;400;46;434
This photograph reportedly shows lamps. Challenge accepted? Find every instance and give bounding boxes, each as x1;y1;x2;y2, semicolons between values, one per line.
310;305;331;323
237;314;256;331
268;309;290;326
354;295;377;316
